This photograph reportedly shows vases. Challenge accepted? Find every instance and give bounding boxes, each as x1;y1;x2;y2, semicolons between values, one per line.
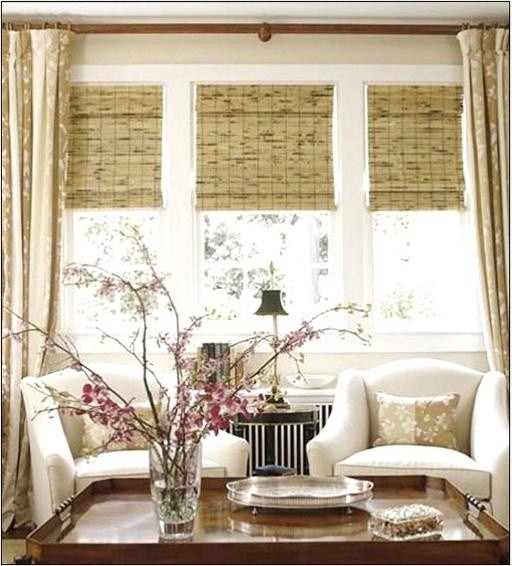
149;437;202;540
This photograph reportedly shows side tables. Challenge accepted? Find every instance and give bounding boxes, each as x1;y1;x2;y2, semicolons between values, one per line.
238;405;317;476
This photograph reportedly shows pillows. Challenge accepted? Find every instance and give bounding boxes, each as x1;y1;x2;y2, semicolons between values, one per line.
372;391;461;450
78;398;169;457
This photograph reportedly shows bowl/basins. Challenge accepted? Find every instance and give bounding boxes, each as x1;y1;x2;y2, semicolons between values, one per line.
283;372;336;389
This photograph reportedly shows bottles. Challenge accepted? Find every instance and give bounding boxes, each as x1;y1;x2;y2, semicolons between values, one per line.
198;340;230;392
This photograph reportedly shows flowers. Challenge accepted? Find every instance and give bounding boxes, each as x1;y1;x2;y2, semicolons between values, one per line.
2;216;372;519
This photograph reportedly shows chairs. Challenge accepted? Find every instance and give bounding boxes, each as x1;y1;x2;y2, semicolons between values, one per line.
14;363;249;564
306;359;509;528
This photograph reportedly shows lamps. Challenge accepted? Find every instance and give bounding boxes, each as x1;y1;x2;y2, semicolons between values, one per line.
254;288;288;410
